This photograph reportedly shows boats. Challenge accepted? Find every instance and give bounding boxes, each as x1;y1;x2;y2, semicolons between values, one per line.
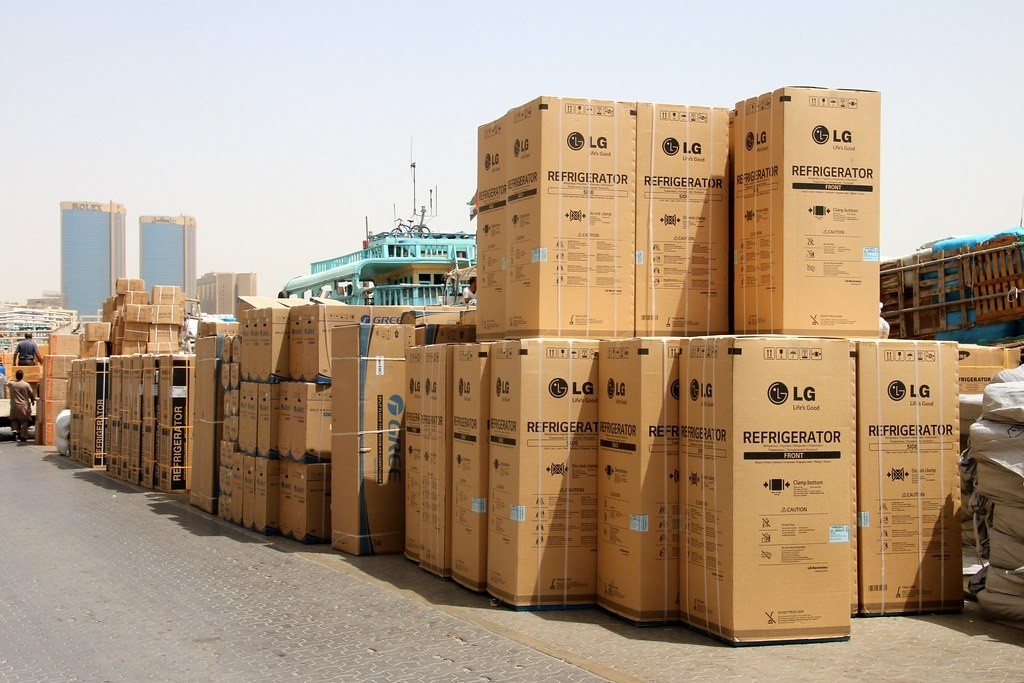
276;132;478;310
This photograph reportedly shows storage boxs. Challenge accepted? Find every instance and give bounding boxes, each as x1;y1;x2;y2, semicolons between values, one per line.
0;86;1022;649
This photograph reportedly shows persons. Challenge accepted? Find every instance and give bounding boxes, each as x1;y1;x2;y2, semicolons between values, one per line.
3;370;35;441
450;278;462;296
13;332;42;366
462;277;477;306
277;288;289;298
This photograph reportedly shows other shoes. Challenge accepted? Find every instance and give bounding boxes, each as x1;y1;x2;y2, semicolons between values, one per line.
12;431;27;442
